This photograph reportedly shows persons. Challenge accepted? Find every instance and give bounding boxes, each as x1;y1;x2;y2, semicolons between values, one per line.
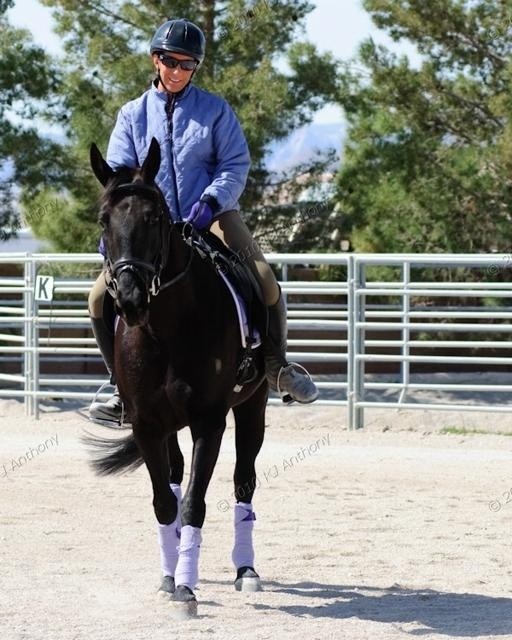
85;17;322;425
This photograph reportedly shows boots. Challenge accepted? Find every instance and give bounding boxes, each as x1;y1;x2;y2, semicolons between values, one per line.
266;282;319;404
89;305;127;424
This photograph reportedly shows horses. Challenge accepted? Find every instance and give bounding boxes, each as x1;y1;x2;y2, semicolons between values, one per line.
79;136;270;622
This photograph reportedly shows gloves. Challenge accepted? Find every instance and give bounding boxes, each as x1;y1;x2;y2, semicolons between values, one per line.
187;193;222;231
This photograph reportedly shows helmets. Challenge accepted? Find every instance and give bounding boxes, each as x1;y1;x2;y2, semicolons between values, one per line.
149;19;207;73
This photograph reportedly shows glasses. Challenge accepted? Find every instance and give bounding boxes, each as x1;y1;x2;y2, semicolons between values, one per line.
156;51;199;71
